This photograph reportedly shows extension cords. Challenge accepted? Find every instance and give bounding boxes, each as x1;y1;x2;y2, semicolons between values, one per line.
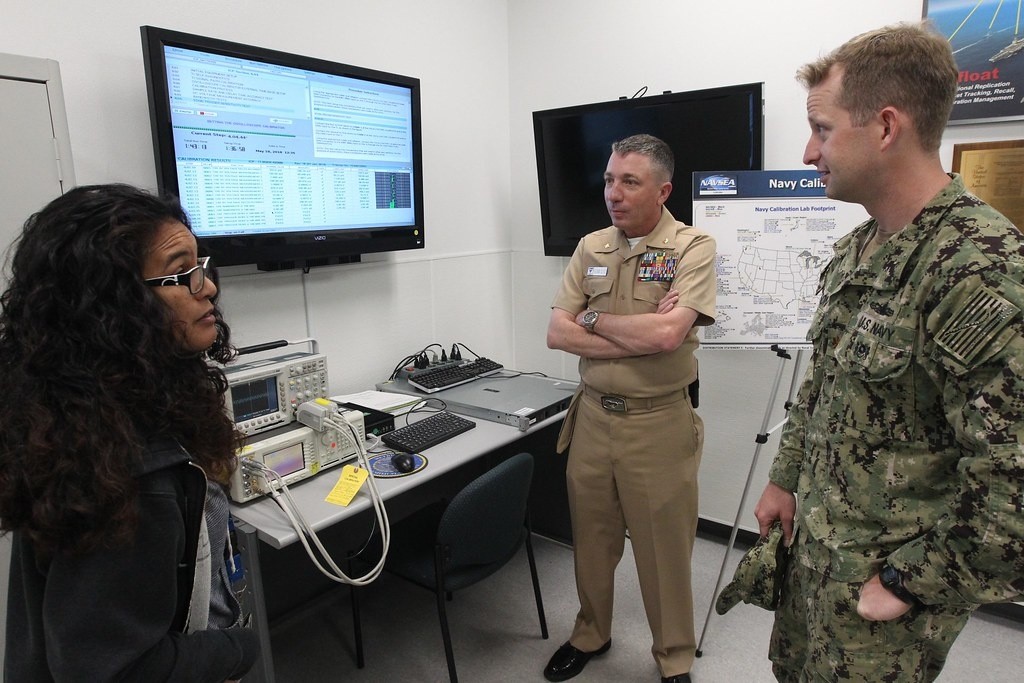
396;358;469;381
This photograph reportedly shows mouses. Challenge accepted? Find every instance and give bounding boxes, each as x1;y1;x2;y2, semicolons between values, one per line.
391;454;414;473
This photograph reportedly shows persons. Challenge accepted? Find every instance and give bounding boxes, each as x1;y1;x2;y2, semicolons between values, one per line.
543;133;717;683
0;182;263;683
755;25;1024;683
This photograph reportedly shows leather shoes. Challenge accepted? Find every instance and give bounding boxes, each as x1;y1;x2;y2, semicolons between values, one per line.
661;671;691;683
544;637;611;682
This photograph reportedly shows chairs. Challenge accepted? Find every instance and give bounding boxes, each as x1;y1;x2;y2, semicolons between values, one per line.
347;452;549;683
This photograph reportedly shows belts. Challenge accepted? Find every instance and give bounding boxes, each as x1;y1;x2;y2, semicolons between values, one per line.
581;380;690;412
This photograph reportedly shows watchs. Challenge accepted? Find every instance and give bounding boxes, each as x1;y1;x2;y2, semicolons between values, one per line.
876;563;918;605
583;310;601;333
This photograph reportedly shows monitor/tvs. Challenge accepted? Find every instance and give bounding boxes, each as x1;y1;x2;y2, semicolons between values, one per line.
231;376;279;424
140;26;425;271
532;82;765;256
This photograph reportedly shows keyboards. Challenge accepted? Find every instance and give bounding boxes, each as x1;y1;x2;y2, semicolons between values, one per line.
409;357;505;393
381;412;477;455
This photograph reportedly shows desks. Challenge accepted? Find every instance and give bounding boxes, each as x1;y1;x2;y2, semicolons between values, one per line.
228;408;574;683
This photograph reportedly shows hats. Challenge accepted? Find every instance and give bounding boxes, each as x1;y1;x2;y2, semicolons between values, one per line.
715;520;785;616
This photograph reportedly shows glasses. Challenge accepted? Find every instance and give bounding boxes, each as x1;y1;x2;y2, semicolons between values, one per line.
145;257;211;295
